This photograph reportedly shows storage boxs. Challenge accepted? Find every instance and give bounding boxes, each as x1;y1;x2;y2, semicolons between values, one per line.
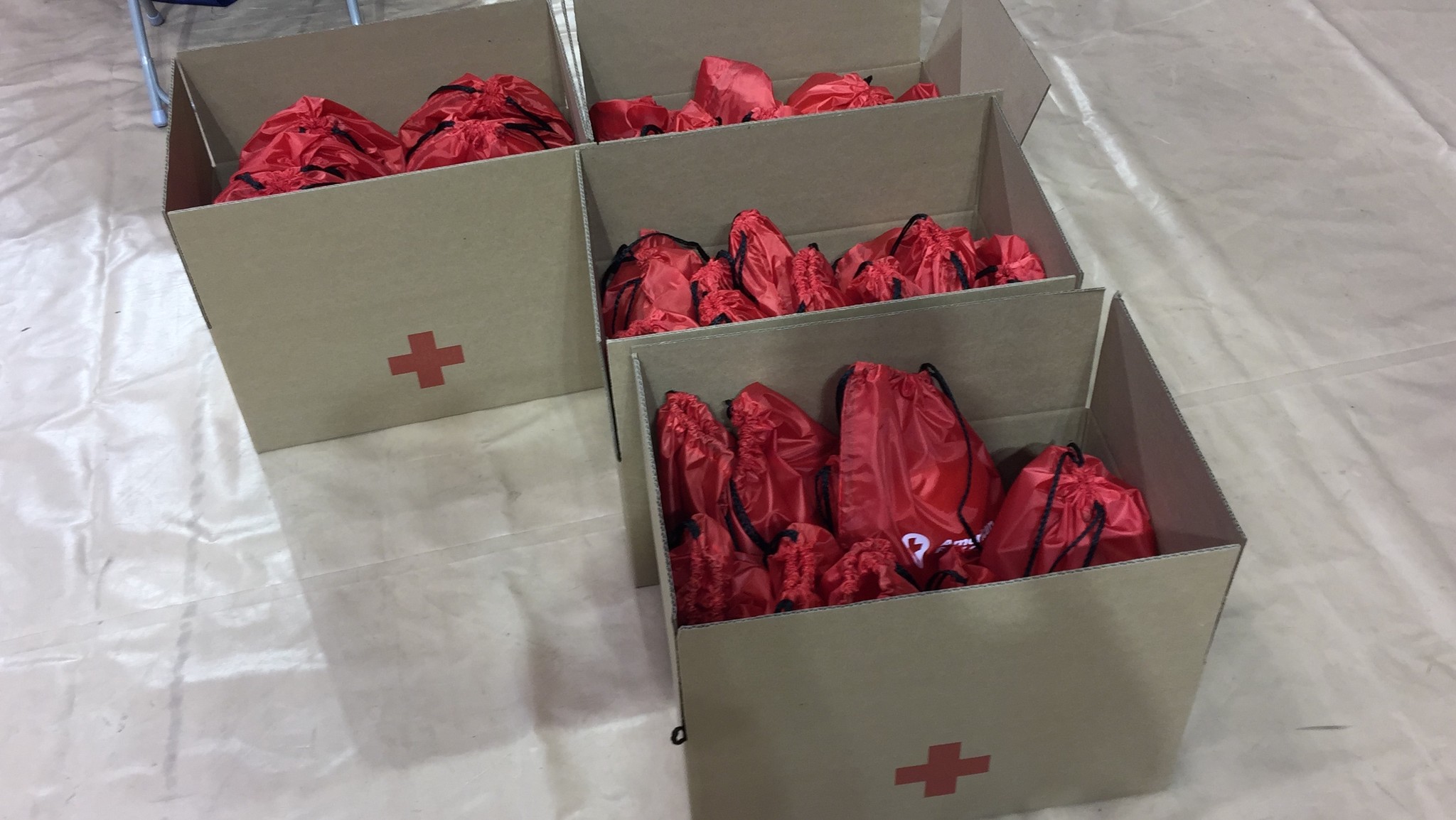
571;0;1054;146
572;93;1086;591
161;1;612;456
629;286;1248;819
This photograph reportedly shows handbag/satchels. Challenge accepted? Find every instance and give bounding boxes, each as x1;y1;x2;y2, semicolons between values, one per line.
651;355;1157;628
584;37;954;160
206;64;582;220
606;172;1045;340
